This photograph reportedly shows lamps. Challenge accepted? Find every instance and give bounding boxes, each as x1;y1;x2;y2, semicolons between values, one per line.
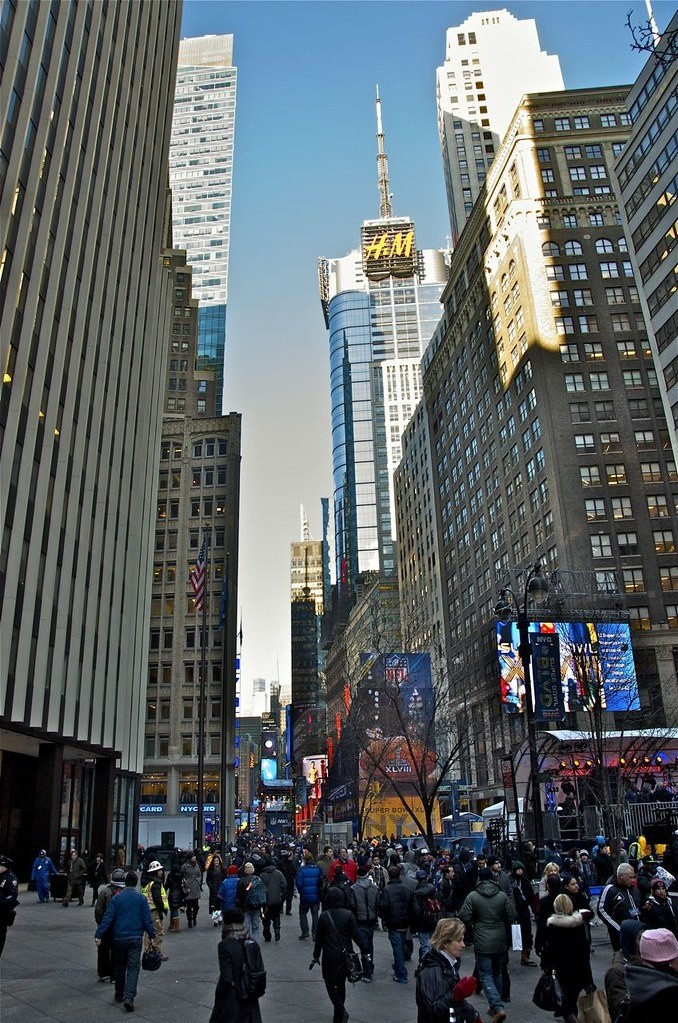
559;756;663;770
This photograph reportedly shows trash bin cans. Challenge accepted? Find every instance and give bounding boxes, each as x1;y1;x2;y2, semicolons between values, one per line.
159;850;174;870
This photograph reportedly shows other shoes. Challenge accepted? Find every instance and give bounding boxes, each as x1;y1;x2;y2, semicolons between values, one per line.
99;973;134;1012
490;1011;507;1023
263;911;317;942
361;956;413;983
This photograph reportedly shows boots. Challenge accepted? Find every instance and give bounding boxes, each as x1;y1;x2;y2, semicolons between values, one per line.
522;949;537;966
167;908;222;933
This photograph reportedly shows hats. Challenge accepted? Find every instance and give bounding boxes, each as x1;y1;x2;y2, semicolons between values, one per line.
650;879;666;895
640;928;678;962
124;872;139;886
112;868;124;880
0;855;13;867
325;886;343;906
357;844;432;880
642;854;658;865
477;849;589;880
227;850;312;874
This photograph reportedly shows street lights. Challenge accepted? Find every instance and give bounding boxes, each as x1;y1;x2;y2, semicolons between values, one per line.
289;756;298;838
494;558;552;878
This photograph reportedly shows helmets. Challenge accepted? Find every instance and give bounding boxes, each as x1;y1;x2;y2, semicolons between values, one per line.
146;861;164;873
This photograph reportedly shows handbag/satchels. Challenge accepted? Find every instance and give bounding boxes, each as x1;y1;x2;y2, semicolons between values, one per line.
579;987;612;1023
511;920;523;951
142;942;161;971
345;950;363;983
532;969;564;1012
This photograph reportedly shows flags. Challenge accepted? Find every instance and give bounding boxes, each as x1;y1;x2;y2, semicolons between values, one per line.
190;538;205;610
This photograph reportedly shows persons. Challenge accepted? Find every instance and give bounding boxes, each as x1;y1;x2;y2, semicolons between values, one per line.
307;762;320;784
117;845;126;869
556;772;678;816
310;888;374;1023
0;855;18;957
458;869;517;1023
540;894;597;1023
88;853;108;906
136;847;206;961
63;851;87;907
210;909;262;1023
605;920;646;1023
416;918;483;1023
94;869;156;1012
624;927;678;1023
508;838;678;967
205;832;514;1002
32;849;59;902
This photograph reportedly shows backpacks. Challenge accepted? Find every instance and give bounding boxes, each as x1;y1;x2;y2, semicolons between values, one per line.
245;876;267;907
238;937;266;1000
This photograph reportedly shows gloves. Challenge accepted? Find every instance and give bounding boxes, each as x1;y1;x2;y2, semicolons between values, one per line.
308;958;321;970
453;976;477;1002
